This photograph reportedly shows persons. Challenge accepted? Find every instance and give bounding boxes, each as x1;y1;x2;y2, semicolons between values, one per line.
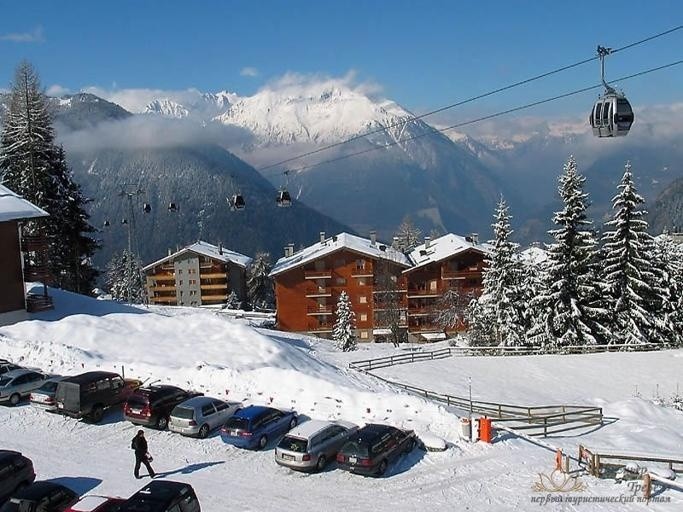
132;430;157;479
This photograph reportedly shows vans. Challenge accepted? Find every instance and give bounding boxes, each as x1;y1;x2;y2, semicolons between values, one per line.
54;370;142;423
274;419;359;472
107;480;200;511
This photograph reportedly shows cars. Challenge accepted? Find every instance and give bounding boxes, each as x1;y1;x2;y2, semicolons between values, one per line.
0;359;41;374
0;480;78;512
336;422;416;478
167;395;243;439
0;368;60;406
122;384;204;429
28;376;72;411
61;495;126;512
0;449;34;505
220;404;298;451
